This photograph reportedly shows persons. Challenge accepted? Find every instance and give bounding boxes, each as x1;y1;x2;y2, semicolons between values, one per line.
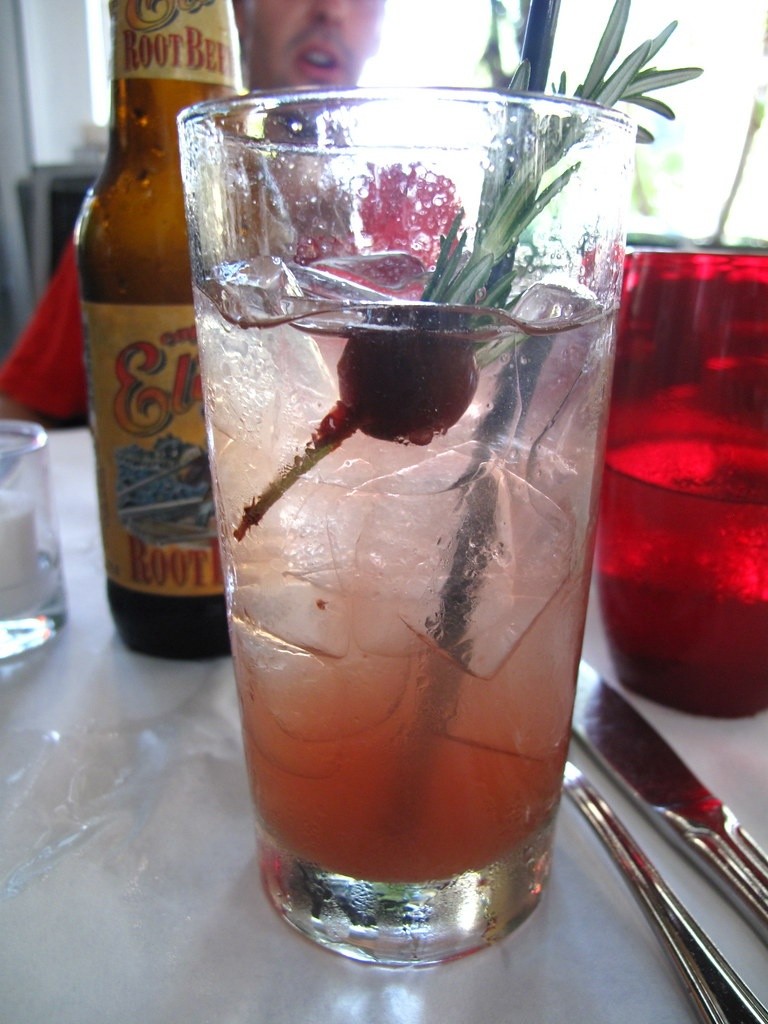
1;0;384;427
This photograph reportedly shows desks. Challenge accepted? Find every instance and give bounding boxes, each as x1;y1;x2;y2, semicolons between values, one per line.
0;428;768;1024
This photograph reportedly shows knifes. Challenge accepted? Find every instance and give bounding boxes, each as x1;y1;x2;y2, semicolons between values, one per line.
569;658;768;947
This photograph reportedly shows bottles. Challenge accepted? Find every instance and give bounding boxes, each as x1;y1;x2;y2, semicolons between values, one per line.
72;0;275;663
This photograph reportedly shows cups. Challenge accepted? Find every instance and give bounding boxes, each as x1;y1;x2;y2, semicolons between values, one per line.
0;418;67;661
579;250;768;720
176;86;638;971
